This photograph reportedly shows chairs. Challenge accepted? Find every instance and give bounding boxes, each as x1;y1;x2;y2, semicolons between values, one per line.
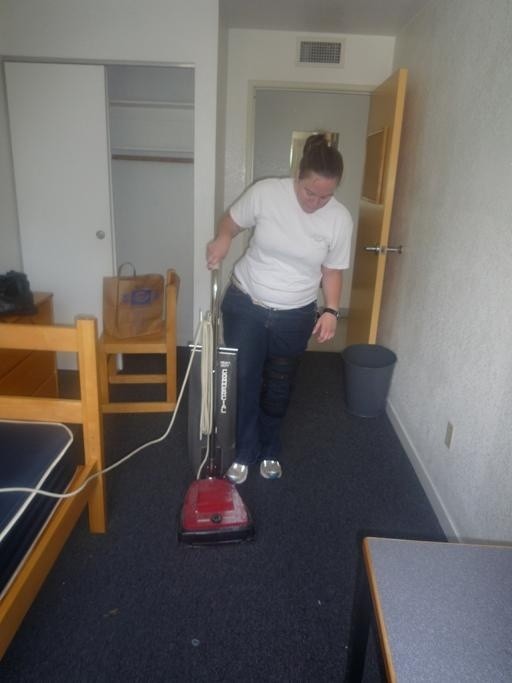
95;268;180;414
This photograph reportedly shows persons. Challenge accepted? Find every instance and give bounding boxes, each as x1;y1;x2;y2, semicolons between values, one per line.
205;134;354;485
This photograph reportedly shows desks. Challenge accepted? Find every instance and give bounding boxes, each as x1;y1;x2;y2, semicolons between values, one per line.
343;536;512;683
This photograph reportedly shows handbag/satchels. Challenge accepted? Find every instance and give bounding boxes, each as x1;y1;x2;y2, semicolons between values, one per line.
102;262;164;340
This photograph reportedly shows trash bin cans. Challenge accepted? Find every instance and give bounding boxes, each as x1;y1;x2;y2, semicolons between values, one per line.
343;343;397;418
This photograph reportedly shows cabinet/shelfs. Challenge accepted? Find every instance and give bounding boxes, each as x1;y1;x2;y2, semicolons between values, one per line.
1;290;59;398
1;54;196;370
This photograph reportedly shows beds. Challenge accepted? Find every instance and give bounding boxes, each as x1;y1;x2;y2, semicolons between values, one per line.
0;316;113;683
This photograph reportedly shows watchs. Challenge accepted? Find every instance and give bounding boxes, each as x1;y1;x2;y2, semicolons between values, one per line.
323;307;340;320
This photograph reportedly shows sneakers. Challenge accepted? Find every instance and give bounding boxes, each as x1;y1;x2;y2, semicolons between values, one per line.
260;460;282;480
223;462;248;484
